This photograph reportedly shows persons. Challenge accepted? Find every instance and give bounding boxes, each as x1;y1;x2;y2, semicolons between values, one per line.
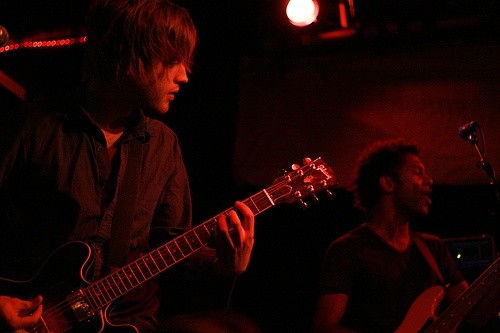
0;0;255;333
315;140;500;333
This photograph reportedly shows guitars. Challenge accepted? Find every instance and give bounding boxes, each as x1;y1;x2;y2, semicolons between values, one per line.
0;152;336;333
393;257;500;333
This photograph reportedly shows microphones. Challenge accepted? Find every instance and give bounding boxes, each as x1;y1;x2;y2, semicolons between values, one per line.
456;121;478;141
0;26;9;47
